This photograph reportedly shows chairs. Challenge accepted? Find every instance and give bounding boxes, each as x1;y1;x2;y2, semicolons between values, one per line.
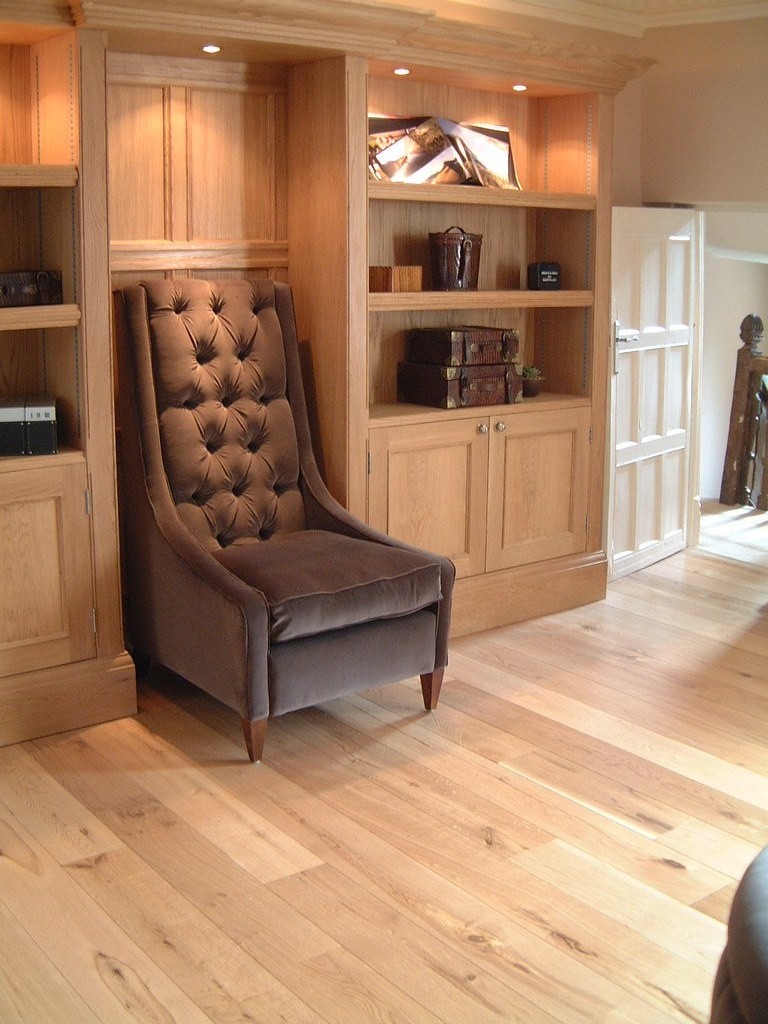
115;280;457;764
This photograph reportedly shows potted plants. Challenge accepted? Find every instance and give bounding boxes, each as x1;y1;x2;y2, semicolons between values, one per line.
522;365;545;397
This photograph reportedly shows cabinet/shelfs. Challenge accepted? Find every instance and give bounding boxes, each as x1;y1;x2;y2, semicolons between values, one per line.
0;23;137;746
279;57;609;638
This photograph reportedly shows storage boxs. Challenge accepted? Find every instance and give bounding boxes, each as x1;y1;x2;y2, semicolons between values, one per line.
398;362;524;409
404;326;522;366
0;271;63;308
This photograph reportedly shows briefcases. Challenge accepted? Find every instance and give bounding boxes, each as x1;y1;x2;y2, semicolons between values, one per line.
406;324;521;366
397;362;523;409
0;270;63;307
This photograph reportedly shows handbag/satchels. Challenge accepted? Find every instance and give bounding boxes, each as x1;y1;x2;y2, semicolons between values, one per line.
428;226;482;291
